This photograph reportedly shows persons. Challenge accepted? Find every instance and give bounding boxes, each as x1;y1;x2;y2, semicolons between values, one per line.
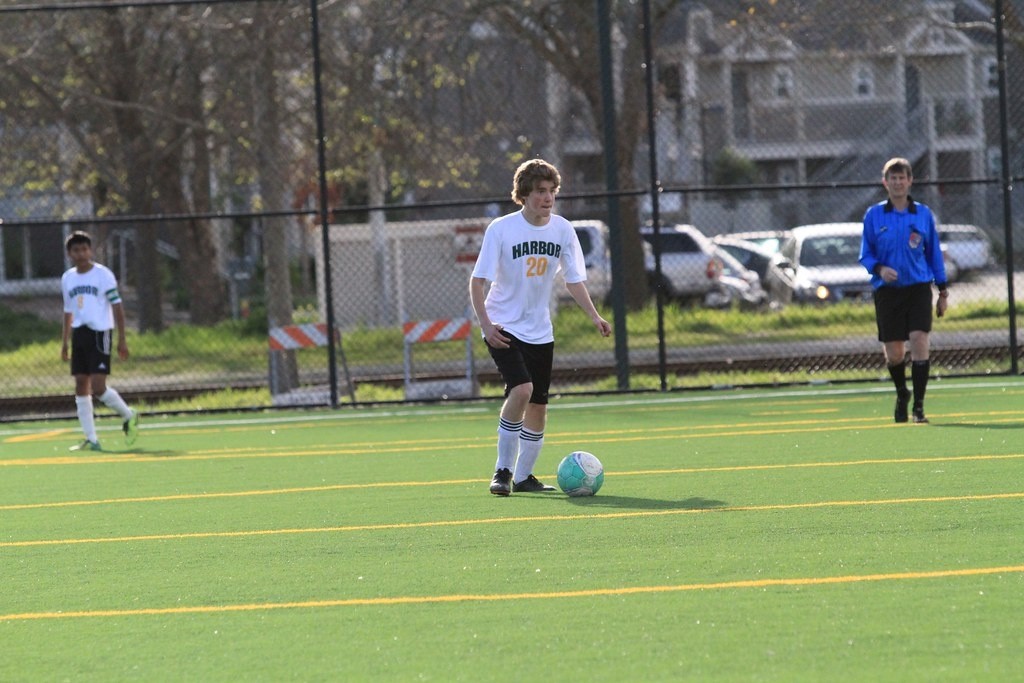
60;232;138;452
858;158;948;424
469;159;611;496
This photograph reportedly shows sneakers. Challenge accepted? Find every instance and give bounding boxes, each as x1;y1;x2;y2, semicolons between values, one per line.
912;407;928;423
512;474;556;492
490;468;513;496
895;389;911;423
122;408;138;446
70;440;101;452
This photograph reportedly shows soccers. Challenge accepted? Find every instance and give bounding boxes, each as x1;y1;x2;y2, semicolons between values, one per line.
555;450;605;497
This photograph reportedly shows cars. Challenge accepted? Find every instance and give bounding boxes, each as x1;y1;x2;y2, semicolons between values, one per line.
769;221;879;302
637;218;778;313
940;224;991;281
547;219;614;312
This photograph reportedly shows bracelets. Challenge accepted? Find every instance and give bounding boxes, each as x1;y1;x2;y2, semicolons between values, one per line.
939;292;948;297
